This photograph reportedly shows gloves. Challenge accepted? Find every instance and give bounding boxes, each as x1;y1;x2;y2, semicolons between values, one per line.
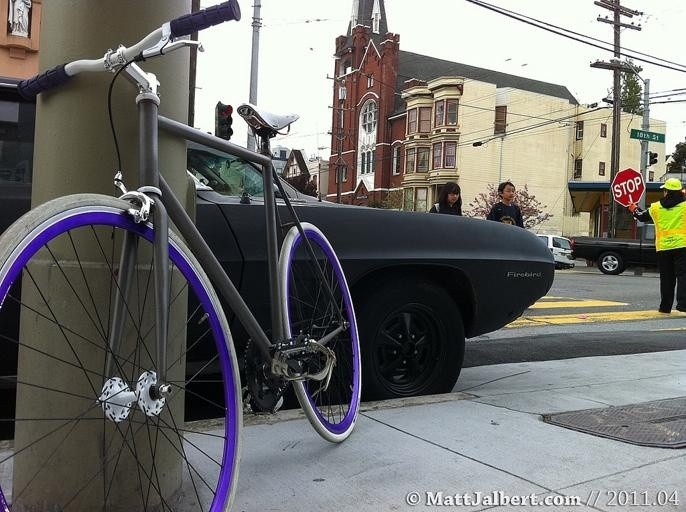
629;202;638;212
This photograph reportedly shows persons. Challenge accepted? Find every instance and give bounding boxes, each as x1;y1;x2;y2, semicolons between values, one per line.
628;178;686;314
8;0;32;38
429;182;462;216
486;182;524;229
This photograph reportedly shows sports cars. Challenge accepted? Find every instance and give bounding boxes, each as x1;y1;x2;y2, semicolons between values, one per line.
0;75;556;430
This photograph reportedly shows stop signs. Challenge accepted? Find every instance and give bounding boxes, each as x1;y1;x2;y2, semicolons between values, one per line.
610;168;646;208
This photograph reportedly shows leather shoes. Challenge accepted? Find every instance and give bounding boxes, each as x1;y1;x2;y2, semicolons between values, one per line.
658;304;685;313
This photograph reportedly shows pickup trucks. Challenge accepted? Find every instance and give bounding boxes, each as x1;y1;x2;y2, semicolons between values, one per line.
569;223;660;277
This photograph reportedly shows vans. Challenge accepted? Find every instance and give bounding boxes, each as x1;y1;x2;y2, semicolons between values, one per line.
533;234;576;271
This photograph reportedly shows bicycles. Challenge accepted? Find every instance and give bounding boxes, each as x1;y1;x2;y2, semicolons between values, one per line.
0;1;367;511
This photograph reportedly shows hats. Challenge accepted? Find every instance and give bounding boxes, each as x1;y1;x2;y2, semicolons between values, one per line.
659;177;682;190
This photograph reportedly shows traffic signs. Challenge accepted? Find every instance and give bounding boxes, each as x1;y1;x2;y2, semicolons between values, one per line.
629;129;666;145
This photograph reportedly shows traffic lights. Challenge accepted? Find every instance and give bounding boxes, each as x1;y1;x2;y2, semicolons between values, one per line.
649;152;659;165
214;101;234;141
